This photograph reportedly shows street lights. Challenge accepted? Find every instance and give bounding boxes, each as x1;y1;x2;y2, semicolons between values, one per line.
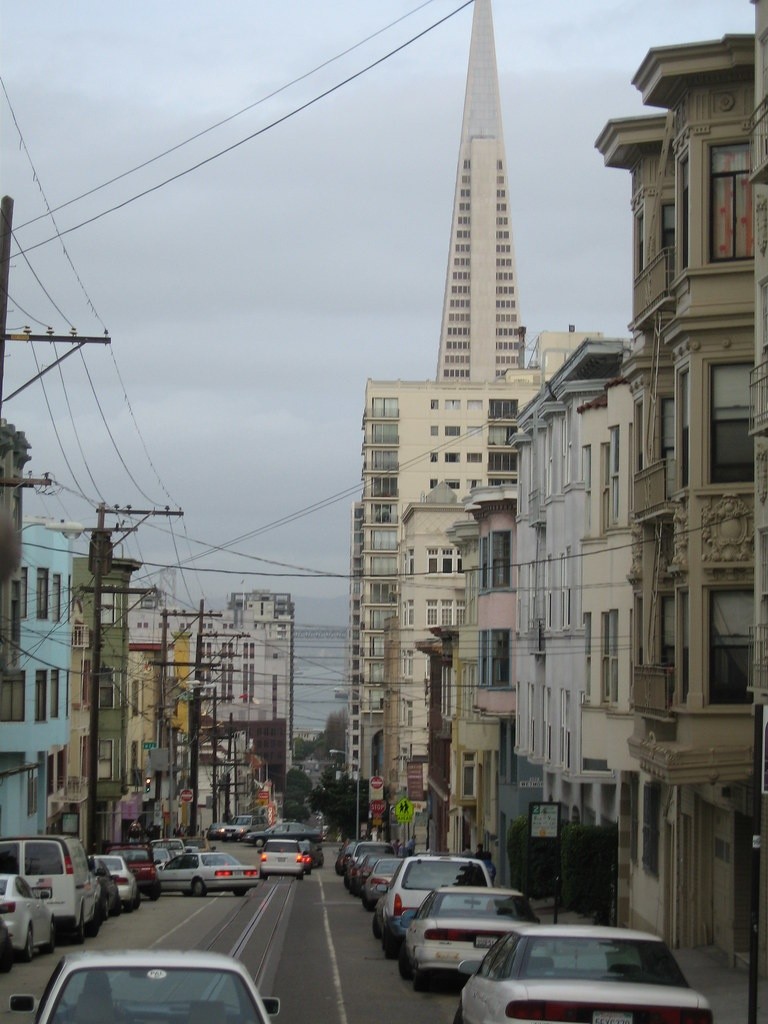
329;750;360;841
334;688;373;779
152;680;200;839
169;684;216;839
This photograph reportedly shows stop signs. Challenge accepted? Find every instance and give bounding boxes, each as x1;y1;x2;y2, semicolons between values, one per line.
371;777;382;788
370;800;385;816
181;790;192;801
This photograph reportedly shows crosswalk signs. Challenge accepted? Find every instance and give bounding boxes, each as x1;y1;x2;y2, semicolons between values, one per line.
395;797;414;822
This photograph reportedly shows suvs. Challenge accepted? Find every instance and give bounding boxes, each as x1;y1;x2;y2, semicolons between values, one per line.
0;835;106;945
106;842;161;901
218;814;269;842
372;852;493;959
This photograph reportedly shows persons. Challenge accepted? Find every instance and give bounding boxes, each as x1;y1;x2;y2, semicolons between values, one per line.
127;818;144;845
406;835;416;856
145;821;157;844
463;844;474;857
64;971;132;1023
392;839;399;856
173;823;187;838
475;843;484;860
483;852;496;881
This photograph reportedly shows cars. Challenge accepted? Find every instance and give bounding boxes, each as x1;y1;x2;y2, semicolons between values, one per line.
257;840;308;880
398;887;551;991
243;822;323;847
157;853;261;897
205;823;227;841
0;874;57;961
94;859;121;921
133;848;171;867
150;839;192;859
299;840;324;876
9;948;280;1024
335;837;405;911
453;925;714;1024
179;837;216;851
94;855;140;913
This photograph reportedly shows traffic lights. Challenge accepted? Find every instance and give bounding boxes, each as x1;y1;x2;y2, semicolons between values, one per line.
146;779;150;792
